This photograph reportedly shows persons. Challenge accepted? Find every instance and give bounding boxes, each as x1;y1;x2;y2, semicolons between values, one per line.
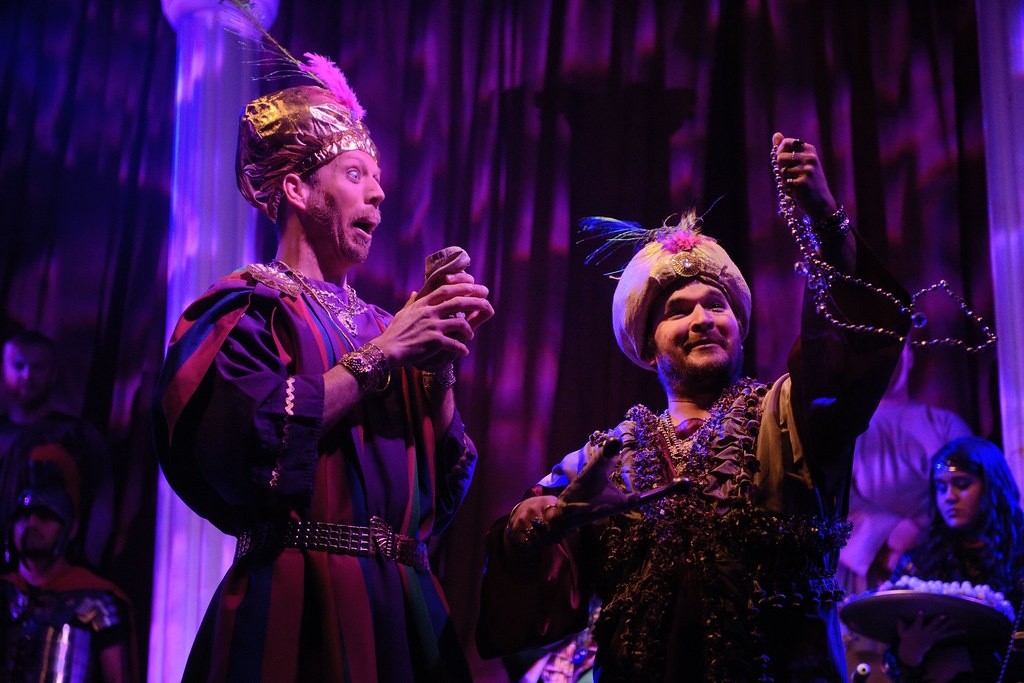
504;130;909;681
0;335;63;485
154;87;495;683
1;486;126;683
886;435;1024;683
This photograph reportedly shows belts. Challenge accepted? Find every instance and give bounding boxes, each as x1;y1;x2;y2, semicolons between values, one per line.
236;517;431;575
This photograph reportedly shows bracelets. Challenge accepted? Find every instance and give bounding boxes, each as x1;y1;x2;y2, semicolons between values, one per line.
422;364;457;390
814;205;852;247
338;342;391;394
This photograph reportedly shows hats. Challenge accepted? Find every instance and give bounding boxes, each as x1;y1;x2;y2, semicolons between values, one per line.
236;86;380;223
613;231;752;371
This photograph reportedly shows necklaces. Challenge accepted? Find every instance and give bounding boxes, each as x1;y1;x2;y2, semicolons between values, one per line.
273;257;358;339
768;148;998;355
657;408;722;469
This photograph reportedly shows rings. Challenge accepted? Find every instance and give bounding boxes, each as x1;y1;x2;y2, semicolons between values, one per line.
522;503;559;548
781;138;804;187
